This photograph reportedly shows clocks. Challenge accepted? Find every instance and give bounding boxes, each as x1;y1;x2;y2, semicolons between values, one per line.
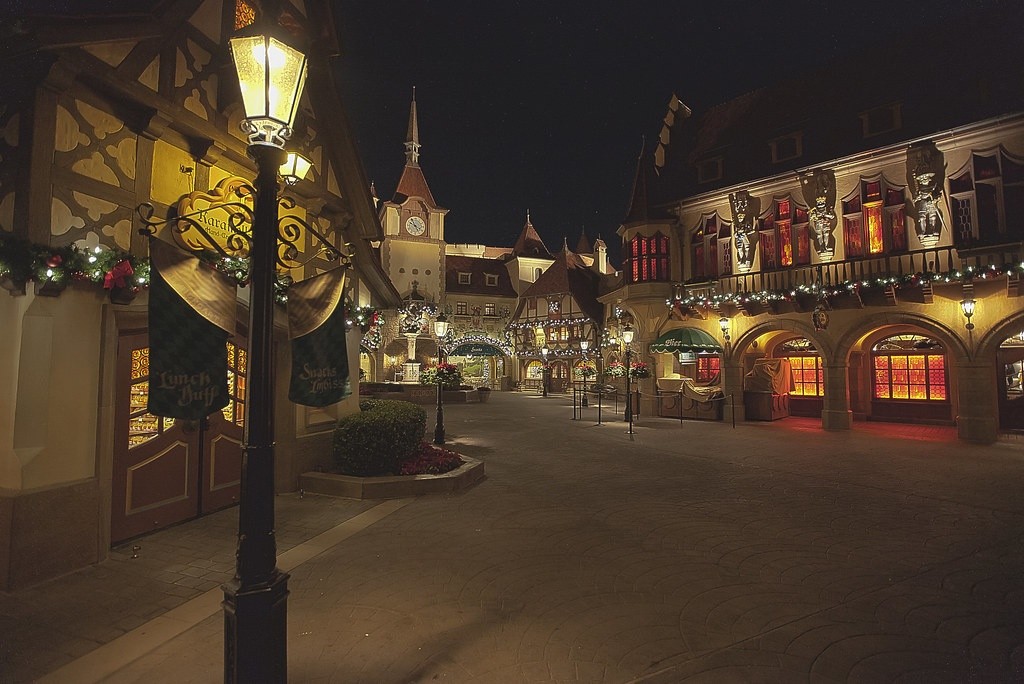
405;215;426;236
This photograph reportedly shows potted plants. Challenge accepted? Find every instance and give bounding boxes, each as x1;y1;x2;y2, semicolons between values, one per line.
477;387;491;402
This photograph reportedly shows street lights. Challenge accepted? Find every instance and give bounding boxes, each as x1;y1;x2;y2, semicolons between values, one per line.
542;346;548;396
622;323;636;435
434;311;450;445
224;18;311;684
580;336;589;407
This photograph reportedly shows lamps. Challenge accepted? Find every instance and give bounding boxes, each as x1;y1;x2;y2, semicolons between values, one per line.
278;128;313;195
959;292;977;332
719;317;729;338
